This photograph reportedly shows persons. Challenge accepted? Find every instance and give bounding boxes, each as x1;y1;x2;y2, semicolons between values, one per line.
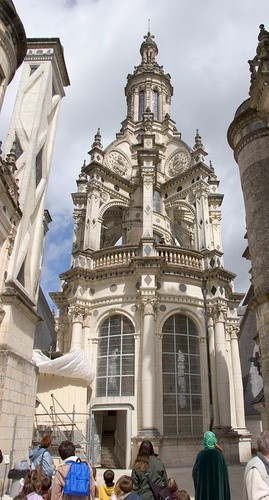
191;431;231;500
243;431;269;500
12;435;190;500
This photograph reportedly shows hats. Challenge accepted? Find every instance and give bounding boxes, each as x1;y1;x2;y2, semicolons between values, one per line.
203;432;217;449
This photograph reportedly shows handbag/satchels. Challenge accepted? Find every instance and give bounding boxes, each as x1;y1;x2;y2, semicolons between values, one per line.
35;450;52;492
150;483;172;500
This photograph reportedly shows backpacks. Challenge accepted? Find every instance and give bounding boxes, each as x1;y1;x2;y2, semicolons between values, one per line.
63;458;90;496
